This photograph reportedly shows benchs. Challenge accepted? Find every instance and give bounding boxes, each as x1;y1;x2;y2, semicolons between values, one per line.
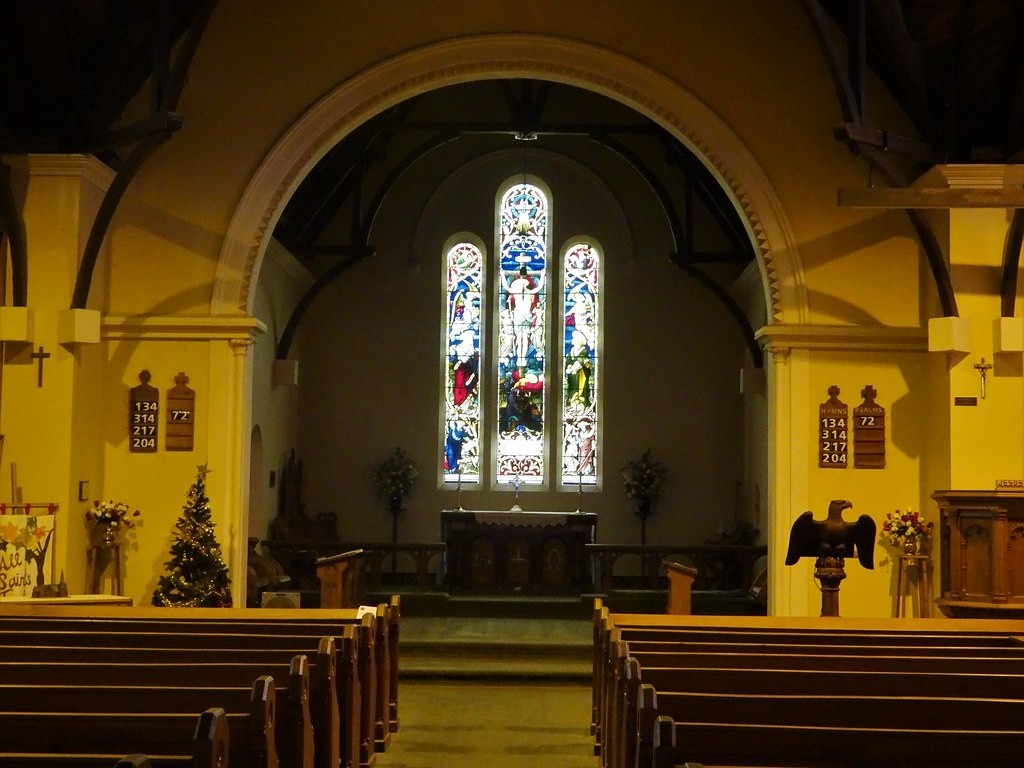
0;593;401;768
585;543;751;596
586;597;1024;768
260;538;448;591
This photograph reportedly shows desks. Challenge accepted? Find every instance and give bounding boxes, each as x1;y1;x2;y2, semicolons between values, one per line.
441;508;598;595
384;507;405;585
897;554;932;619
92;544;122;595
632;510;656;543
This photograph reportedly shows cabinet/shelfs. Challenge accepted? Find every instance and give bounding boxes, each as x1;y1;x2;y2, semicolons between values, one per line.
931;490;1024;620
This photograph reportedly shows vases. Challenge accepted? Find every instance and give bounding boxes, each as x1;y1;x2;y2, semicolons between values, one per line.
388;492;402;509
100;527;118;544
638;495;651;514
902;536;919;556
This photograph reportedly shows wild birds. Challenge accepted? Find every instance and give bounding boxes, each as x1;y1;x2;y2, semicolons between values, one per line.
784;499;877;571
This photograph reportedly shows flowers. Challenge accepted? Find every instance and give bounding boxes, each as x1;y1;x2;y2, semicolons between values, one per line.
620;448;673;503
881;508;934;546
367;446;420;499
85;500;141;531
